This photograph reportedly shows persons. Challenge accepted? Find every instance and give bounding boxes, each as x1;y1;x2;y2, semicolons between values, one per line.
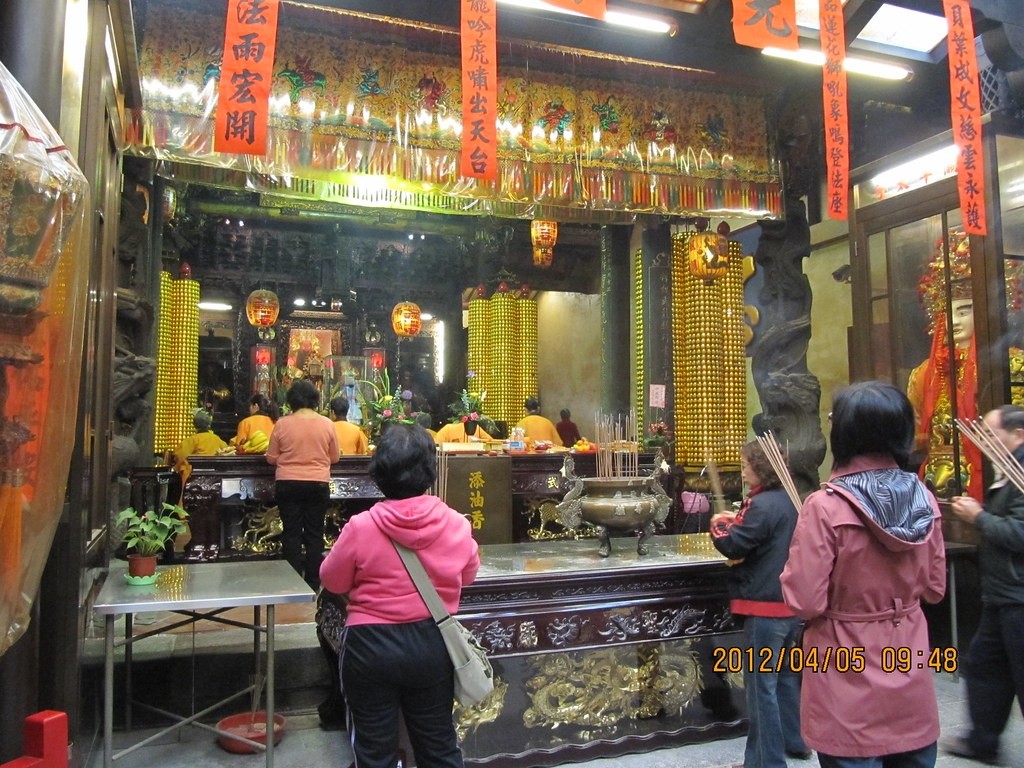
171;412;229;519
432;400;492;443
266;382;340;591
319;421;480;768
556;408;581;447
235;395;279;446
513;398;564;447
904;234;1024;497
779;379;946;768
328;397;369;455
710;440;813;768
941;407;1024;758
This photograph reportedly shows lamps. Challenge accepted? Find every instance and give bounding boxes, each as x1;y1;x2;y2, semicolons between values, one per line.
761;0;949;81
499;0;679;38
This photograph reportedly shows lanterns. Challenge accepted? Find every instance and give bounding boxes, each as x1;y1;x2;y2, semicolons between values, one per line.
530;220;557;268
246;290;279;328
392;301;421;338
688;232;728;285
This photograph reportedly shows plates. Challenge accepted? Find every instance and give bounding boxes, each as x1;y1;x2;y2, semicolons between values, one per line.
507;448;595;454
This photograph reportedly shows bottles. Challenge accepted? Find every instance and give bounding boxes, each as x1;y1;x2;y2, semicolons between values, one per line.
509;427;524;450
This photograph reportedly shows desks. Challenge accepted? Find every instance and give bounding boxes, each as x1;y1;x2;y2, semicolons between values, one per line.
91;561;317;768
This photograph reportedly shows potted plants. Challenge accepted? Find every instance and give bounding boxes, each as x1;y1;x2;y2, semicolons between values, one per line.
116;503;190;576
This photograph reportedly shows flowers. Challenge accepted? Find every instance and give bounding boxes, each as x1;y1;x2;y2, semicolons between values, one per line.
355;374;415;426
448;388;501;434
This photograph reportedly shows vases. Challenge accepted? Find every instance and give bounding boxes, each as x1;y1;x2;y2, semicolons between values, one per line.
465;421;478;436
371;424;384;441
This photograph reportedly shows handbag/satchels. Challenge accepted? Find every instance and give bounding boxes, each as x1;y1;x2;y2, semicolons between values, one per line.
437;615;495;707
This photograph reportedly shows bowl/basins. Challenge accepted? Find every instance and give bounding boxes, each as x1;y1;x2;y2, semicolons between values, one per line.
215;710;286;753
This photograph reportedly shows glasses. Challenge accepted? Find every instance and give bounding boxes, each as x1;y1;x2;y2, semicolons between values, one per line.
827;412;834;424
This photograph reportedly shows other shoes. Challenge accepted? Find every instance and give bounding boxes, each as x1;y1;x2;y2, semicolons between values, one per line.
785;750;813;759
944;737;994;764
